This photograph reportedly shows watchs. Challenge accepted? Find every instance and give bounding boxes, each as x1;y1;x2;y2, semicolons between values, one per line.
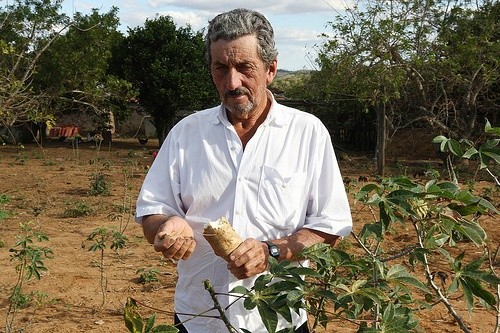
260;240;281;266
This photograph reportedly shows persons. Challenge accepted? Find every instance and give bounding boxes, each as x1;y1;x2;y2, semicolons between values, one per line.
133;8;354;333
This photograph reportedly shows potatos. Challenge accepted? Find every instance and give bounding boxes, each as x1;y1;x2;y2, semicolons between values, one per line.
202;215;244;264
